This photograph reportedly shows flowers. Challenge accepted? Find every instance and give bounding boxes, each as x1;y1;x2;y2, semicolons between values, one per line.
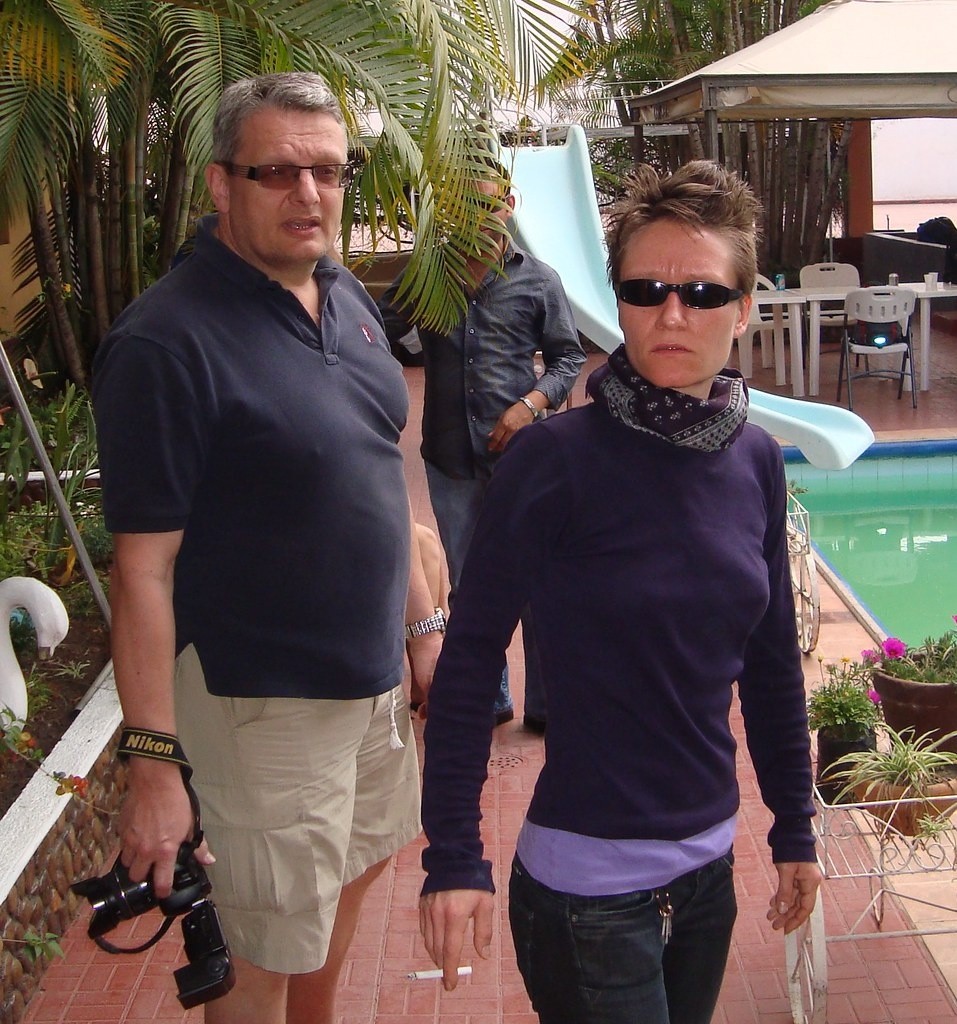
807;658;883;743
860;614;957;677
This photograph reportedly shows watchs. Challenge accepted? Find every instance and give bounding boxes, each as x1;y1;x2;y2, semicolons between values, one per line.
405;605;446;640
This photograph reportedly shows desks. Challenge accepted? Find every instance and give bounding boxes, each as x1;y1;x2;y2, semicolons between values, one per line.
735;282;957;395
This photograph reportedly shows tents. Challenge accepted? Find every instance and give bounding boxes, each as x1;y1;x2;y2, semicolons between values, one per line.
627;0;957;182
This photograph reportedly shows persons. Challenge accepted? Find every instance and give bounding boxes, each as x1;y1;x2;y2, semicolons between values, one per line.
418;160;825;1024
88;73;443;1024
404;521;449;726
374;155;587;734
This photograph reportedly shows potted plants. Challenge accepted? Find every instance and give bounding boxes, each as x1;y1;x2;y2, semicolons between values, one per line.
817;721;957;838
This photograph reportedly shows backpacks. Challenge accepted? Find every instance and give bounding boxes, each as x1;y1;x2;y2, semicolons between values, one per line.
918;215;957;281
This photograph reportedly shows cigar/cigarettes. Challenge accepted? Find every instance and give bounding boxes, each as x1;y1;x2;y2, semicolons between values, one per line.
406;965;473;982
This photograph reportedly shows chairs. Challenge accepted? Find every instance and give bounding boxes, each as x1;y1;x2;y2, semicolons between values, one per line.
737;272;784;392
839;284;919;415
798;263;862;392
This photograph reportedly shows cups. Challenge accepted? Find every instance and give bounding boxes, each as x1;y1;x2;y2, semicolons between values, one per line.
924;275;933;286
929;273;939;286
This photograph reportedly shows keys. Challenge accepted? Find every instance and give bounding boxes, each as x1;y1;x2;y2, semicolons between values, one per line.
653;887;674;944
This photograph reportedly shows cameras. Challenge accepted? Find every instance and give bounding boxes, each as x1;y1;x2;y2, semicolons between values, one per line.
68;839;237;1010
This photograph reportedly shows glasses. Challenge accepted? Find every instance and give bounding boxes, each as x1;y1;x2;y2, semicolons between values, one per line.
213;160;353;190
451;193;510;213
612;277;744;309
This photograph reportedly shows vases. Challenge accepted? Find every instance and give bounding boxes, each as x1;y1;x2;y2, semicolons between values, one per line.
872;651;957;753
814;726;877;802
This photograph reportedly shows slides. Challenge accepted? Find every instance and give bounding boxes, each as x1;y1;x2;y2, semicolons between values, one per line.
485;121;876;472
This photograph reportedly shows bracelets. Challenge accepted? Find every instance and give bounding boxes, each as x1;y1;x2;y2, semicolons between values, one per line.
519;397;540;418
